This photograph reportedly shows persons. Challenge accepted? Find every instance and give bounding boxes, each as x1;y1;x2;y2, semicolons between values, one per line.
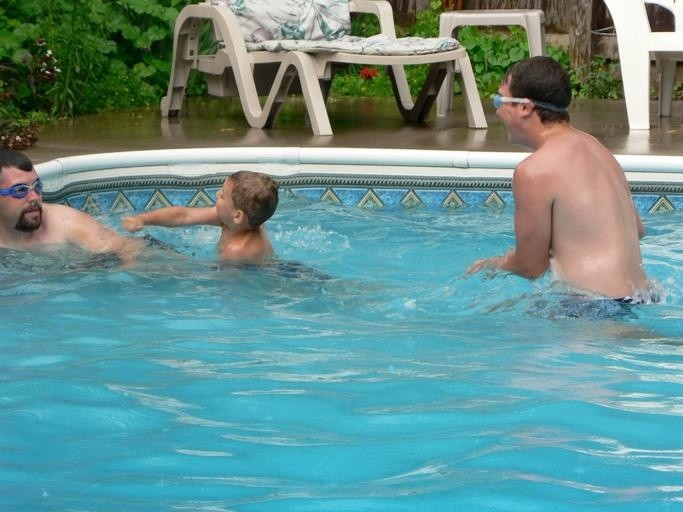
460;56;663;322
117;168;402;304
0;145;157;273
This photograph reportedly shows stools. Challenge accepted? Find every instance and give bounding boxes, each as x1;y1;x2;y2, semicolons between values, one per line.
435;10;546;118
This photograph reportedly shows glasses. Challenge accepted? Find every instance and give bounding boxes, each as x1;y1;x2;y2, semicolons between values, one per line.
0;180;42;198
492;94;534;109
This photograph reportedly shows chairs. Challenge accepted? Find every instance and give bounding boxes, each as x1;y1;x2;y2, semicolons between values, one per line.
160;0;487;137
